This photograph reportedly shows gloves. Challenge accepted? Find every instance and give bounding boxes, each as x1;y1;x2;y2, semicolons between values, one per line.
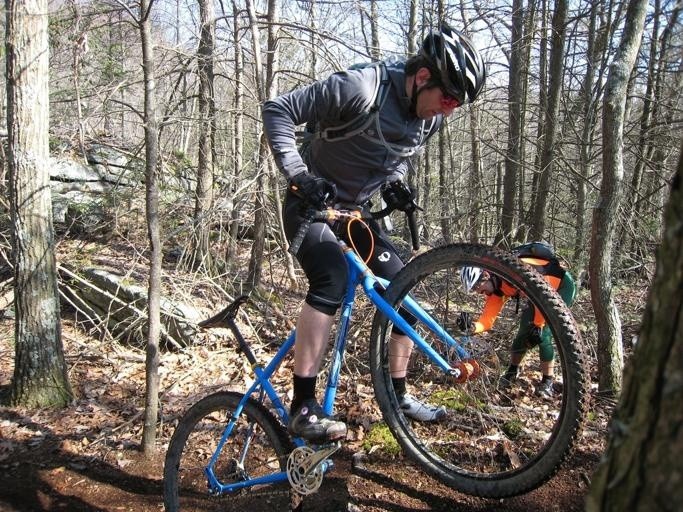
288;170;338;212
456;312;472;331
380;180;419;216
526;323;542;347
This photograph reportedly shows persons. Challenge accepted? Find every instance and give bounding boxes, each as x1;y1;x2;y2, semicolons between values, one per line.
456;242;579;399
260;21;486;447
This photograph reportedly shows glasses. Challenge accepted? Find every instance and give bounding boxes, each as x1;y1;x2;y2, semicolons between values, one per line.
425;62;461;110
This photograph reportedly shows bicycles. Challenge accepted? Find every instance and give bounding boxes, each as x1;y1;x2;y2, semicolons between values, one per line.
163;185;592;512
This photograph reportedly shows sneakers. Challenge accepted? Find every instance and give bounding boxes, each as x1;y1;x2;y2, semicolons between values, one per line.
535;377;554;401
397;390;448;423
287;398;349;442
497;366;520;387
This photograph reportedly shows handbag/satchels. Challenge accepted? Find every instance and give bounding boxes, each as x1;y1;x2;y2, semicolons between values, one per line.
510;241;556;260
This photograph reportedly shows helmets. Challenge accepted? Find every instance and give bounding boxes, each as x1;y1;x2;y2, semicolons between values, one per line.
419;20;486;104
459;265;484;295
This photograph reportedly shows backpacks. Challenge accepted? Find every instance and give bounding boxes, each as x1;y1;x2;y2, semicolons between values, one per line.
304;62;437;143
